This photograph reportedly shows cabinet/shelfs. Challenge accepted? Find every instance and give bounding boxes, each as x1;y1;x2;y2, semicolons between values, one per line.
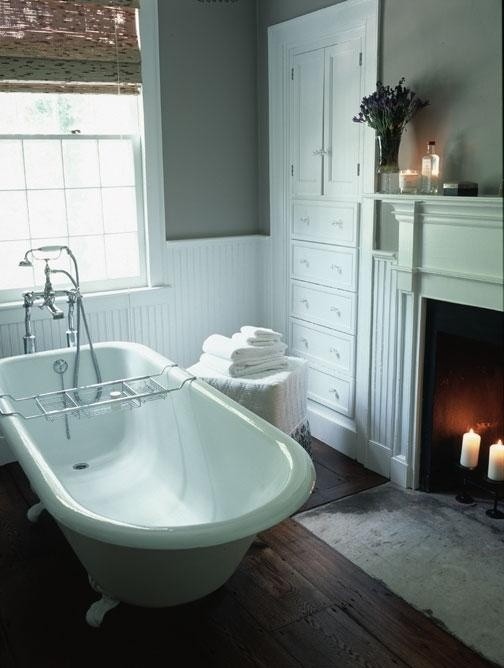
266;1;386;464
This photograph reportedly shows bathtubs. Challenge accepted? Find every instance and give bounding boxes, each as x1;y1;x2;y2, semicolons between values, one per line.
0;341;318;610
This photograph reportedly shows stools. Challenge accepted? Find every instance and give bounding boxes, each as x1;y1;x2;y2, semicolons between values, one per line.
184;347;314;469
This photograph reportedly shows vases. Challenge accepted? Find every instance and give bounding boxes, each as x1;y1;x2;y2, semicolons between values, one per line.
371;132;403;176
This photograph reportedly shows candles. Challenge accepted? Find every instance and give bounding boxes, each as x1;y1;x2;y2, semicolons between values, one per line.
488;439;504;483
395;168;418;194
458;427;482;471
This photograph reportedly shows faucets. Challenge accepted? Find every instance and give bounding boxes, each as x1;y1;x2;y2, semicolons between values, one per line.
37;299;65;319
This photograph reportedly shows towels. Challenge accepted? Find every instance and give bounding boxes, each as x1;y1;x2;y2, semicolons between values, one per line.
194;321;288;379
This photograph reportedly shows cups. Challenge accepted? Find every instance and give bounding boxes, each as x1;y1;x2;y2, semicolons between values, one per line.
399;171;418;194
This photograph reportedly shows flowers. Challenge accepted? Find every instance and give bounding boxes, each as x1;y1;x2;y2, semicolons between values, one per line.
350;74;434;175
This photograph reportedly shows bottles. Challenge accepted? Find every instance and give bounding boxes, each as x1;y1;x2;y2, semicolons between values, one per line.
422;140;441;193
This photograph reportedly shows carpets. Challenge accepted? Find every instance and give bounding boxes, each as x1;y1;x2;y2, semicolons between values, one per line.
288;476;504;668
